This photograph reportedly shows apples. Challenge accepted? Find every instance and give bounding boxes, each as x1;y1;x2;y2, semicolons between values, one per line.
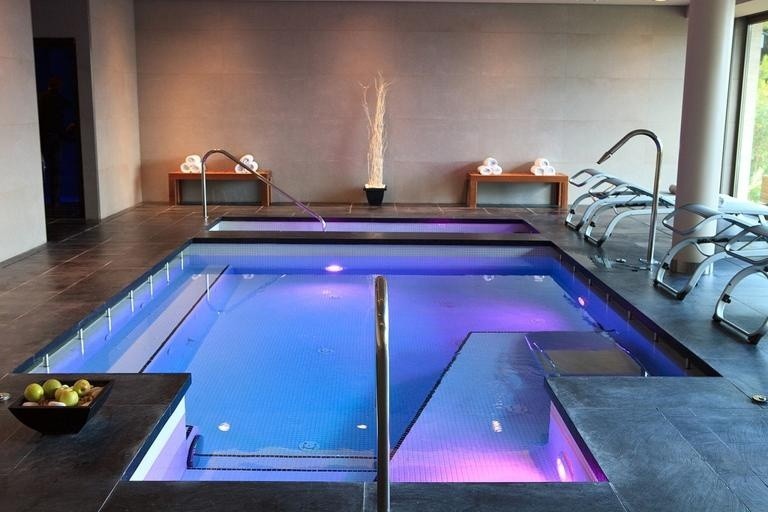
23;377;91;406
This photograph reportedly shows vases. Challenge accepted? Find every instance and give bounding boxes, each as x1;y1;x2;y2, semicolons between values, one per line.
363;183;387;206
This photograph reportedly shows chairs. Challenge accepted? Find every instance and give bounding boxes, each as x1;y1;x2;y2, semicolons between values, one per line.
565;168;768;344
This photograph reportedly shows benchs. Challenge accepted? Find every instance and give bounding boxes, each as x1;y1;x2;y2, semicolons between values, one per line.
466;172;568;208
168;169;272;207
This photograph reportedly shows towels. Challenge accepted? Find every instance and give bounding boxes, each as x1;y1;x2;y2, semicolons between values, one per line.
234;154;258;174
531;158;555;176
180;154;206;174
477;158;502;175
